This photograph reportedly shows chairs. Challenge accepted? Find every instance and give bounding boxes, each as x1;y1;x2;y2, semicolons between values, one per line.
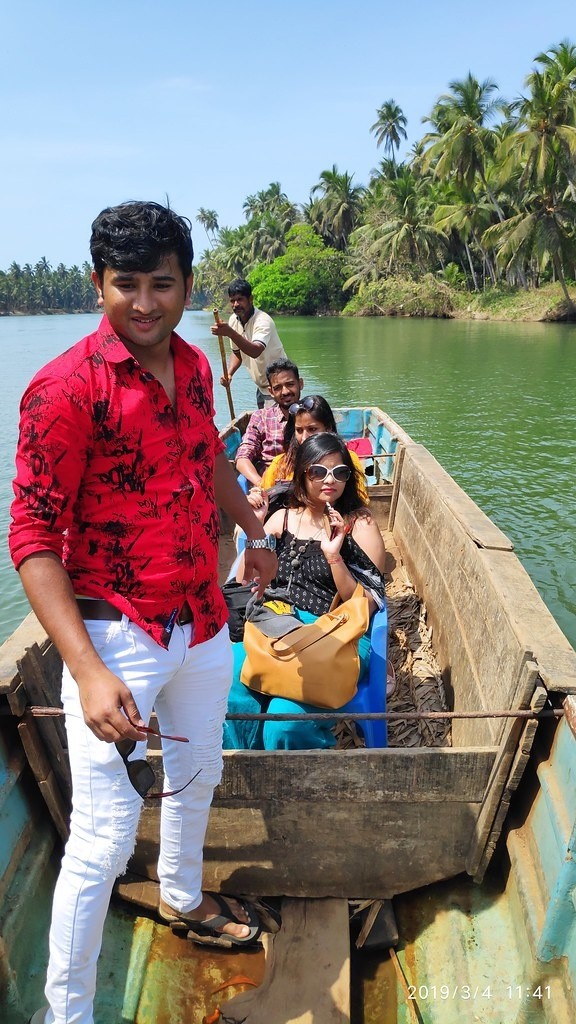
234;472;389;748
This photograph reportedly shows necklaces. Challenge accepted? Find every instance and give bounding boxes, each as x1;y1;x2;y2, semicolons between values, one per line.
289;509;324;567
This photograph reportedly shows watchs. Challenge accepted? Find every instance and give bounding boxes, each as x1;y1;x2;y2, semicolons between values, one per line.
244;532;277;554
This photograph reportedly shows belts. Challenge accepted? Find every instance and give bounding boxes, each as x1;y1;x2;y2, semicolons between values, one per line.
72;592;197;627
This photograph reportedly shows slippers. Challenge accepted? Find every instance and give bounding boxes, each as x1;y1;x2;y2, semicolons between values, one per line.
152;892;261;945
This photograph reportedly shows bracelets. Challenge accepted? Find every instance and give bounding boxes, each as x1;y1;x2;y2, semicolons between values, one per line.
326;557;344;565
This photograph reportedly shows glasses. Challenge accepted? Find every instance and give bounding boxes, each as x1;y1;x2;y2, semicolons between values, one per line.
303;461;353;483
286;395;317;414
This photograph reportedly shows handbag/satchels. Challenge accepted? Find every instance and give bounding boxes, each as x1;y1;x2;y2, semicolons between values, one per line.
221;579;249;642
239;579;370;710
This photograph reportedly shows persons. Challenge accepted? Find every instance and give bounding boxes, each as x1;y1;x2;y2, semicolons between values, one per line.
257;393;371;506
229;429;387;747
232;356;305;489
210;278;289;410
8;198;279;1024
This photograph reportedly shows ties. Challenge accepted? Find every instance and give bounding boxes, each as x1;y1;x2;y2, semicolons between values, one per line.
114;719;203;801
